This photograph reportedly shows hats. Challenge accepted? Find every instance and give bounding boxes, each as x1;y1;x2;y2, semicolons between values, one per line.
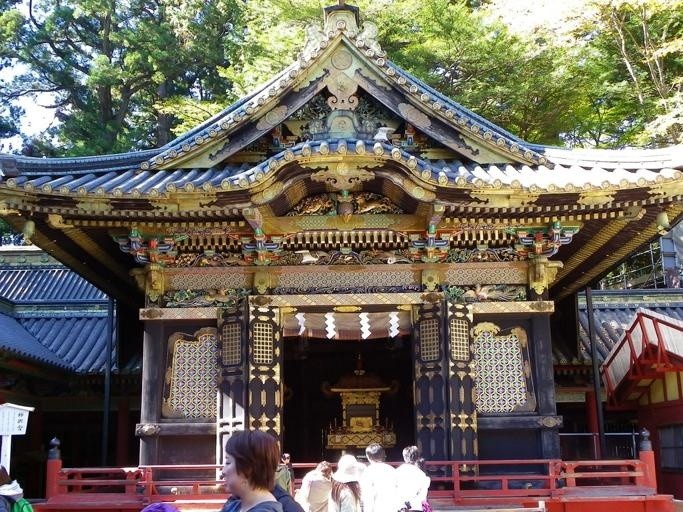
332;455;367;483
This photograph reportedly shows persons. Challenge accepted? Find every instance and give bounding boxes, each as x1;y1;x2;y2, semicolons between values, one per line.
0;464;33;512
219;429;432;512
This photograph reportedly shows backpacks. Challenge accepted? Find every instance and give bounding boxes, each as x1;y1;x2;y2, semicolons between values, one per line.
3;496;32;512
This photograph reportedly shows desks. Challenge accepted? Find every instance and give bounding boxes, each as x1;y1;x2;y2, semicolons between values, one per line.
325;433;396;449
